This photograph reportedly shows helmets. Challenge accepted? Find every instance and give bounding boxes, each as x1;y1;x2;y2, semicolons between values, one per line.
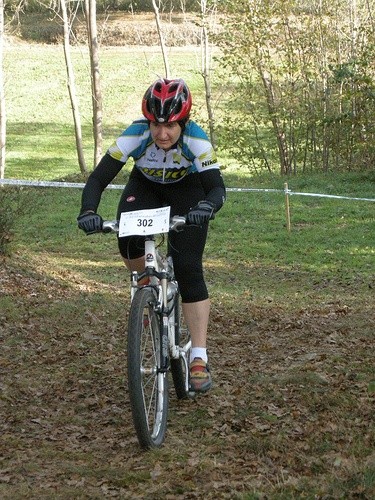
142;78;192;123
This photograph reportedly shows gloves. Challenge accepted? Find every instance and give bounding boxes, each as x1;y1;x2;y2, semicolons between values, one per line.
77;210;102;233
187;201;216;225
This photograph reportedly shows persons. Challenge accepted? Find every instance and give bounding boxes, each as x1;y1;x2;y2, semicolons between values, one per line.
77;78;226;396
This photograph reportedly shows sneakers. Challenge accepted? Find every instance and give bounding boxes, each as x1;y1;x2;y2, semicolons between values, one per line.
137;276;161;327
188;357;211;392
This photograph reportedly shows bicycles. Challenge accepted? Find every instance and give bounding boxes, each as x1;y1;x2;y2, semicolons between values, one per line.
86;206;215;452
